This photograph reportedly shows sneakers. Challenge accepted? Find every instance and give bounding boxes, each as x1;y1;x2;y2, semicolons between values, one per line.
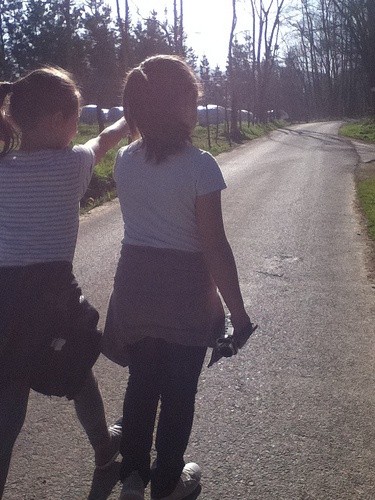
159;463;201;500
119;471;144;500
95;416;123;470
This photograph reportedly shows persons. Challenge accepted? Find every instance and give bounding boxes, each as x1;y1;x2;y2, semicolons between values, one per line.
100;54;251;500
1;64;130;500
